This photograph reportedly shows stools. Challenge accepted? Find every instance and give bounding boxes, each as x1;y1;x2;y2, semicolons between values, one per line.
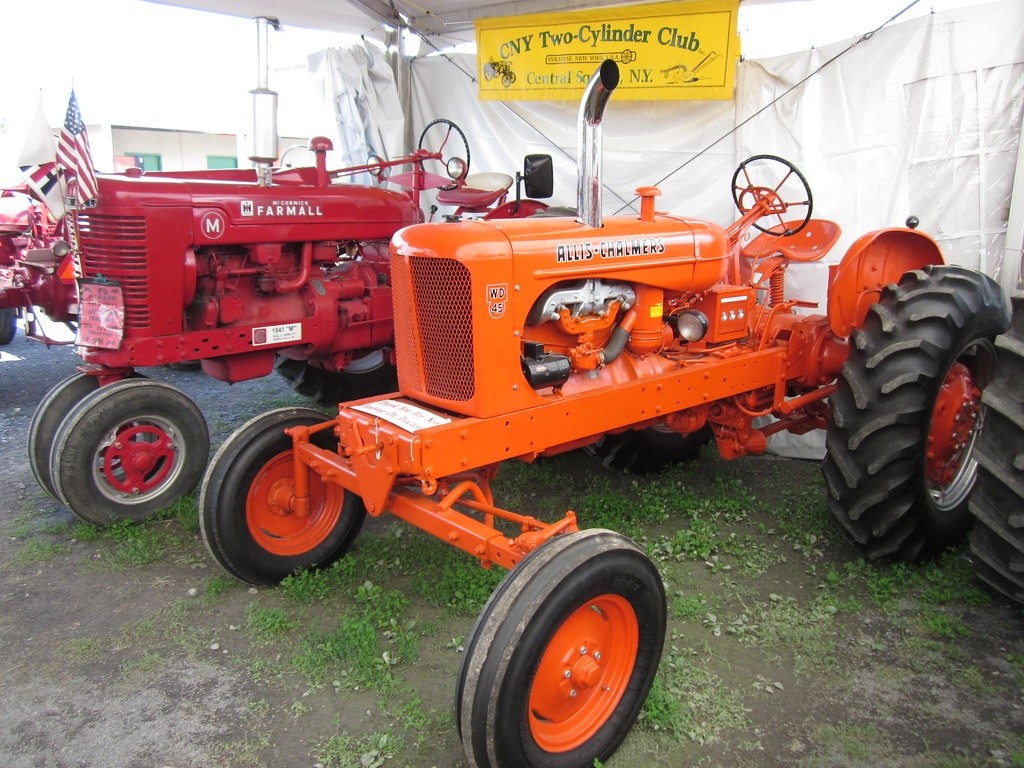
436;171;514;220
741;219;841;313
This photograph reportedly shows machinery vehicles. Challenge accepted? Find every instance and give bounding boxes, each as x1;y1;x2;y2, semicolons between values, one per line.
195;58;1013;768
0;154;103;345
27;115;556;531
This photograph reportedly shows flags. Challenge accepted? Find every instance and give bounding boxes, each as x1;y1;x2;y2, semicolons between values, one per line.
56;89;99;205
